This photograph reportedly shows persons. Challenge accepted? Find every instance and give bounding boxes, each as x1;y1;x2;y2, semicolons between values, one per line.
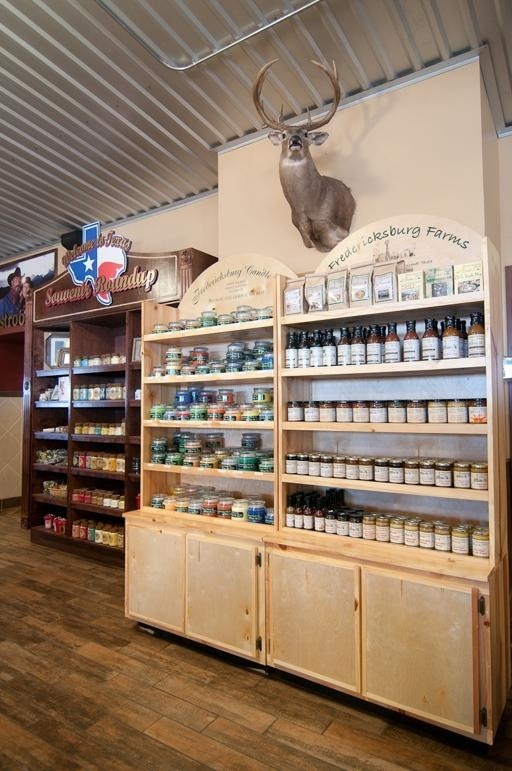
16;275;34;312
0;266;23;317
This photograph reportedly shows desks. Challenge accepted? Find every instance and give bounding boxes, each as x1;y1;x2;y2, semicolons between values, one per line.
23;237;217;565
122;251;294;675
268;212;507;752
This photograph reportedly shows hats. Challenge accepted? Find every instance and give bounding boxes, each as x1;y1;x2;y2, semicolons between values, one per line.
23;277;31;284
7;267;26;286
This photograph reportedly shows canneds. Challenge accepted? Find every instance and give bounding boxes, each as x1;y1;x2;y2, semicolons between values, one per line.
287;398;487;423
286;452;488;490
73;352;126;548
286;504;489;558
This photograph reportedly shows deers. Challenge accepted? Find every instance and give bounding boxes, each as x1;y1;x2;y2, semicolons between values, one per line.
252;58;356;253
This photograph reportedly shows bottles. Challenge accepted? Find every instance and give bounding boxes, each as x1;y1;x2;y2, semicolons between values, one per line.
150;431;274;472
150;340;274;377
44;513;69;534
285;450;489;490
71;451;125;471
285;312;485;368
153;305;272;333
73;418;125;435
71;519;124;548
151;481;274;524
285;490;490;558
150;385;273;421
73;383;123;400
73;353;126;366
286;398;488;423
72;487;124;511
